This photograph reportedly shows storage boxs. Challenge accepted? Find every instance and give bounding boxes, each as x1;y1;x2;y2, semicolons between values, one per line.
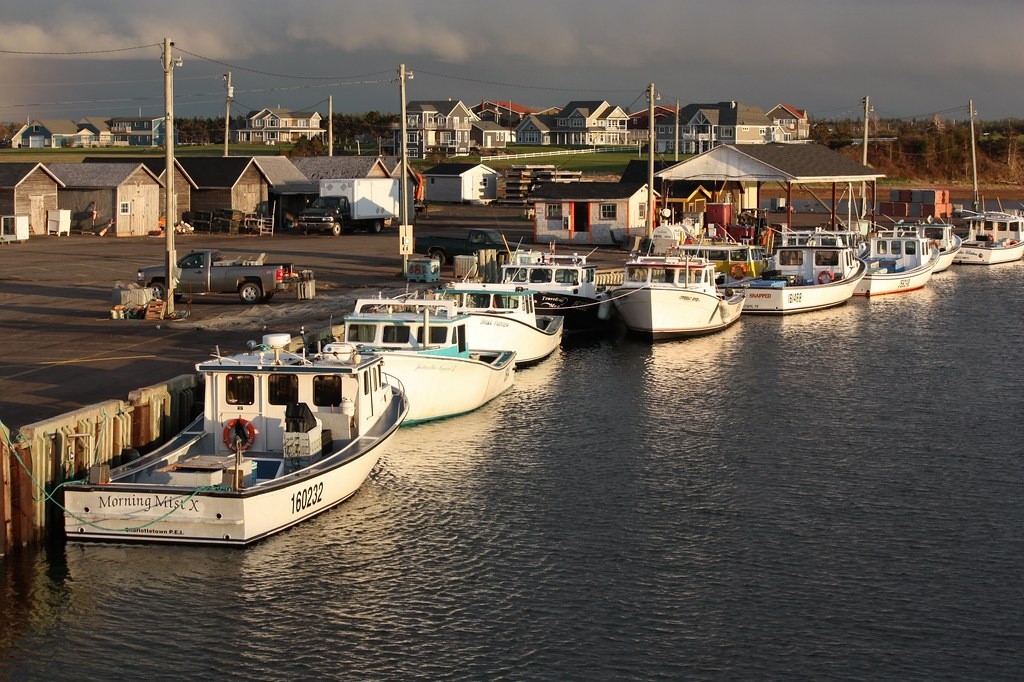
212;259;264;266
169;419;333;487
878;188;964;218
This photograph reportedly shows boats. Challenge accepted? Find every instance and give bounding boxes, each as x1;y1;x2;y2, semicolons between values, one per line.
435;282;564;365
911;223;964;273
500;251;609;335
323;299;516;426
611;254;747;342
852;236;940;296
952;211;1024;265
65;330;410;549
717;231;867;314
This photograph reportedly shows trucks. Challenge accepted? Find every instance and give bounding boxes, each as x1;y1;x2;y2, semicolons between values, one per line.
299;178;401;237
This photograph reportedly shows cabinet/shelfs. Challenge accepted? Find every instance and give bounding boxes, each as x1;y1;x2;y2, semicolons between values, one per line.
46;210;71;236
0;215;29;244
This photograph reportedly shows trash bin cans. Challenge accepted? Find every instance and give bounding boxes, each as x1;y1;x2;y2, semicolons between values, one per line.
296;270;315;299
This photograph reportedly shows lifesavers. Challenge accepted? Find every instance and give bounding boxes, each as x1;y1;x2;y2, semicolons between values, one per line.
984;234;994;246
730;263;747;280
222;419;255;452
818;270;835;284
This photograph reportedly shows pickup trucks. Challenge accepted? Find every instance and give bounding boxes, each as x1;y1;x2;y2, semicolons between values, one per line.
415;228;532;268
138;248;294;304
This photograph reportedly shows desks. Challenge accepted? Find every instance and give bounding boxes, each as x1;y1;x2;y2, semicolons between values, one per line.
295;275;316;299
112;288;155;308
406;258;442;282
454;255;480;278
